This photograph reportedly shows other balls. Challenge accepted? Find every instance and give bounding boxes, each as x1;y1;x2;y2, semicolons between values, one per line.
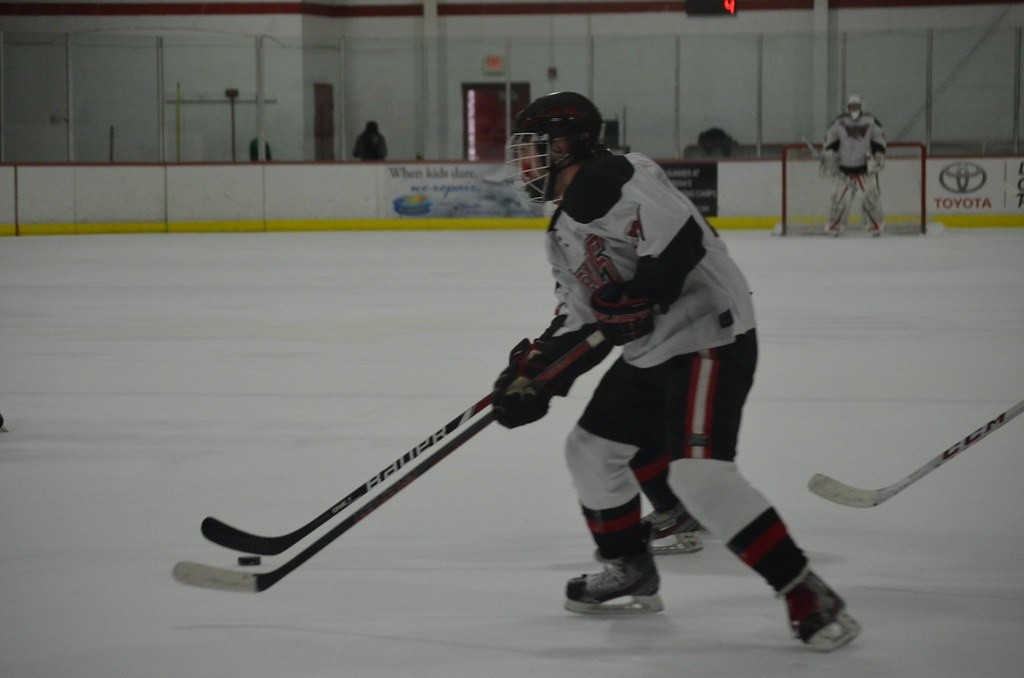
238;555;261;565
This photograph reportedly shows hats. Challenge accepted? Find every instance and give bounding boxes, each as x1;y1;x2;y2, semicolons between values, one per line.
366;121;377;133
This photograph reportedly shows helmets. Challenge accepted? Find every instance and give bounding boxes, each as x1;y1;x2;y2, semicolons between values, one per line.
846;94;866;113
503;90;603;205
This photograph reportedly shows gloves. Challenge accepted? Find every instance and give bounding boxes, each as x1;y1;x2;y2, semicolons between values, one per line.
818;149;833;178
491;338;555;428
875;152;885;170
590;279;657;347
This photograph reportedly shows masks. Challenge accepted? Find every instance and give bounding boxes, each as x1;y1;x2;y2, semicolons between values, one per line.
850;109;861;120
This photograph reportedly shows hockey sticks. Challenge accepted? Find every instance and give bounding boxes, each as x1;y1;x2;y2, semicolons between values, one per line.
169;289;660;591
802;387;1024;510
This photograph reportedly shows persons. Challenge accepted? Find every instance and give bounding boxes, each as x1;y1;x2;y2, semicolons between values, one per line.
489;148;760;552
351;120;390;164
481;87;864;655
822;93;888;235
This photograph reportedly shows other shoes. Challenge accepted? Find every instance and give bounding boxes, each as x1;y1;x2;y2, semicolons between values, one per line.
786;569;861;650
640;499;705;554
562;522;663;615
